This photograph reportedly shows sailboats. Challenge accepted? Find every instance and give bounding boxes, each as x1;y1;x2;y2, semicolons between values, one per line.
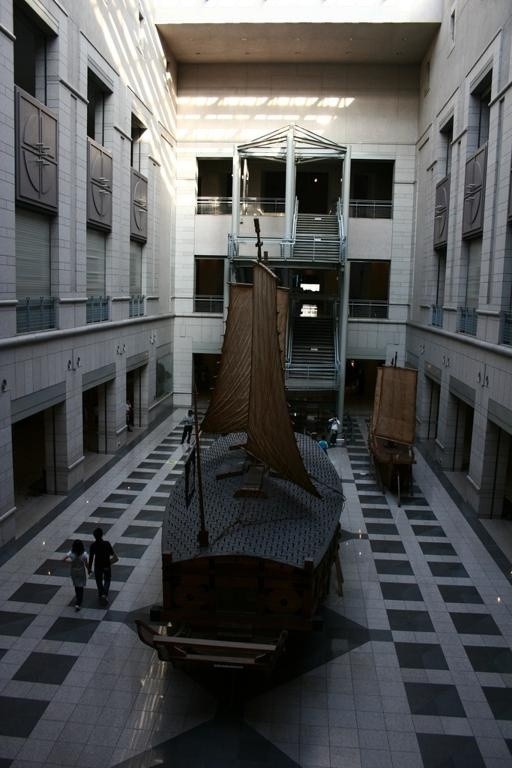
366;350;420;507
132;215;346;694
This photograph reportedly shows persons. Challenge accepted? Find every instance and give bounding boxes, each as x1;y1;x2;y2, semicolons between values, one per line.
318;435;329;457
329;415;340;446
180;409;194;444
64;539;93;610
88;528;119;605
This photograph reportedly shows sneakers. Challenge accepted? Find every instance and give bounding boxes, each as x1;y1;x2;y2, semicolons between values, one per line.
101;595;109;602
75;605;80;610
